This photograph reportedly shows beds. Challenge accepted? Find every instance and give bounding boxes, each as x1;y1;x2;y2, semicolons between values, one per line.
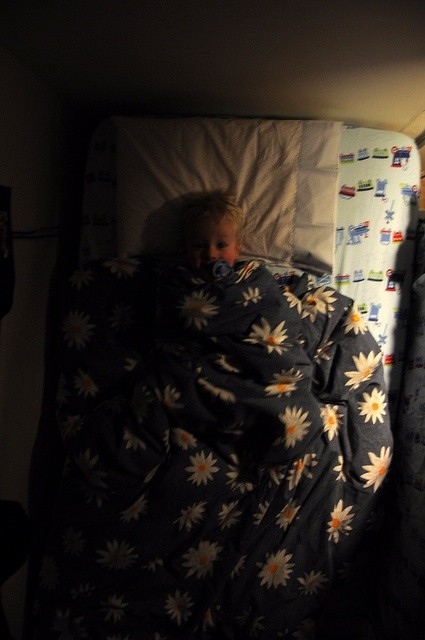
20;116;423;640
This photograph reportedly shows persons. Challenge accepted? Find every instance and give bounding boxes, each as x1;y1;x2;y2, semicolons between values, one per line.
180;191;242;269
